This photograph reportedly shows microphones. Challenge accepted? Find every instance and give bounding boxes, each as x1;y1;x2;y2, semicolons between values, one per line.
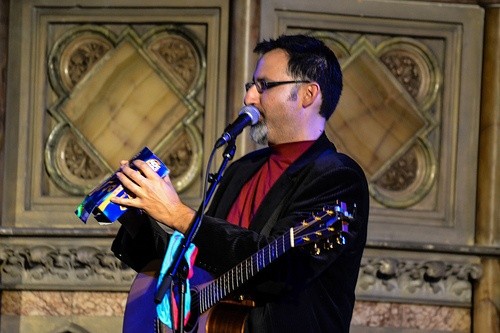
214;105;260;148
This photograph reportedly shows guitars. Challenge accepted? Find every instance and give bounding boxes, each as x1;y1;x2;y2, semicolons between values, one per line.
121;198;356;333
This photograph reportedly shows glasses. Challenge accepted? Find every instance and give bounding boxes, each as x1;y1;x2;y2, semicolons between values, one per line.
245;79;312;94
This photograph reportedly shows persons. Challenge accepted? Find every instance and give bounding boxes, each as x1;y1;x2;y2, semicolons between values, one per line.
111;33;369;333
